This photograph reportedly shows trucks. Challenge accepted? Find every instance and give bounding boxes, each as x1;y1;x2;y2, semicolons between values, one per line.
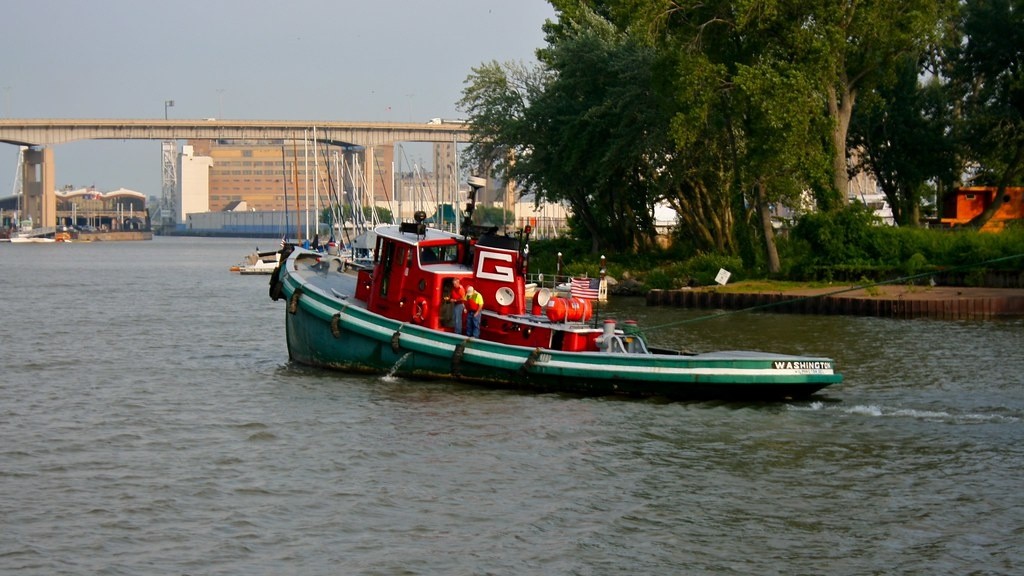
425;117;477;125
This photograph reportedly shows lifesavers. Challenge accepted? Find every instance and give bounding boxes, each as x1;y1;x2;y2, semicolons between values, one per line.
411;296;428;323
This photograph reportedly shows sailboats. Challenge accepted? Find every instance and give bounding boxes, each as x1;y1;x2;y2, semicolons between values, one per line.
231;127;457;275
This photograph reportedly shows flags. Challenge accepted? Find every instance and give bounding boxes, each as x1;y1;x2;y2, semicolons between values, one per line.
166;100;174;106
571;278;599;300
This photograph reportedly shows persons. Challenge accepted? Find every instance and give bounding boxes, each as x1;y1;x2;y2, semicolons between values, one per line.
464;286;483;338
444;279;465;334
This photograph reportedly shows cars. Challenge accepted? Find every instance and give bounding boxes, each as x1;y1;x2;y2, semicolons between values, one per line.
58;223;111;233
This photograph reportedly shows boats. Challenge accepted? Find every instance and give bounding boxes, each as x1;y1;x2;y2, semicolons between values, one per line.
269;179;844;402
10;237;56;243
523;282;611;306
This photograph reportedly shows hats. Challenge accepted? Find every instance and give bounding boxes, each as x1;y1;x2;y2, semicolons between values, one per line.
467;286;474;296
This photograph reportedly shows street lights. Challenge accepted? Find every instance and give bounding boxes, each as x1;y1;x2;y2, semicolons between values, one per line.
164;99;173;120
